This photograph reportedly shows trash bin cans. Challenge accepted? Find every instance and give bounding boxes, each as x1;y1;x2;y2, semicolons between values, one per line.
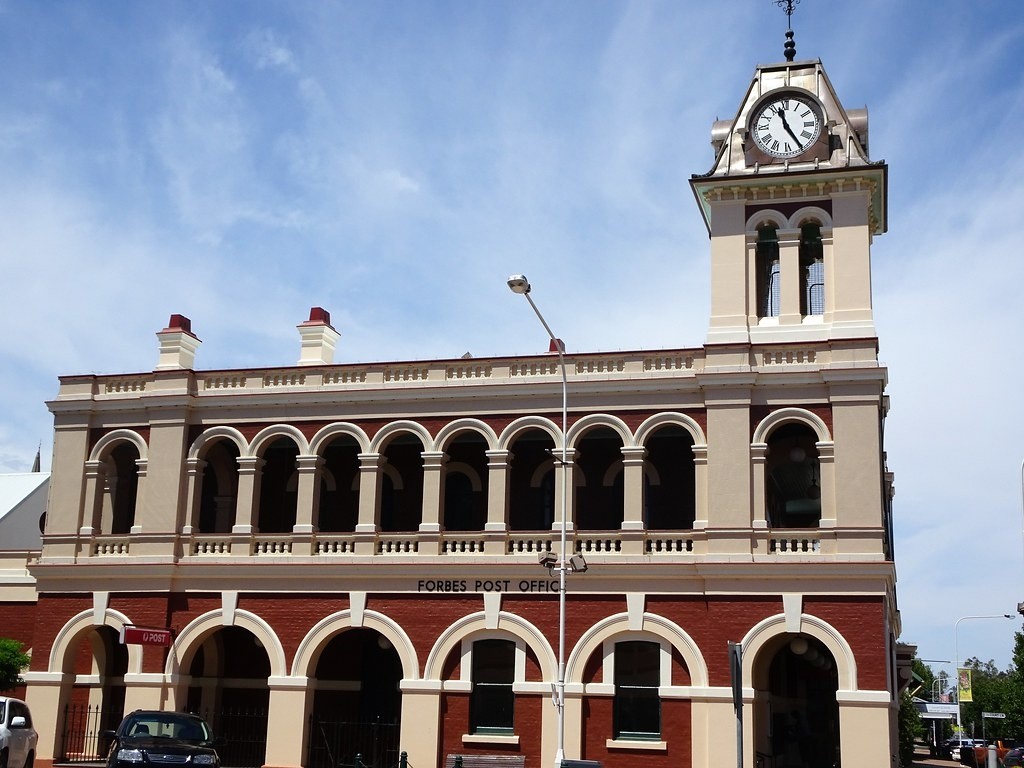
986;744;998;768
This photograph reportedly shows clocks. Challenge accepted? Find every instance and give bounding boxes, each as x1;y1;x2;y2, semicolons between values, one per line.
750;97;822;158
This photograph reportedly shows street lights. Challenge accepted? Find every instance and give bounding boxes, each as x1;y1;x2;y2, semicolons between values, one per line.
931;677;959;754
938;660;973;761
507;273;568;768
954;614;1016;764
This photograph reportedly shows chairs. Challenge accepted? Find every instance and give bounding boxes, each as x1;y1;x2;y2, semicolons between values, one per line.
134;725;149;735
178;729;192;738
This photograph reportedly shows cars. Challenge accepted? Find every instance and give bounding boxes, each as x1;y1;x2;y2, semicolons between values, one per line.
0;694;40;768
103;709;221;768
938;737;1024;768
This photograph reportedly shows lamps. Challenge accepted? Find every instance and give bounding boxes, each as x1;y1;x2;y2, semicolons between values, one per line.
808;464;820;499
789;436;806;462
803;642;832;671
791;634;808;654
